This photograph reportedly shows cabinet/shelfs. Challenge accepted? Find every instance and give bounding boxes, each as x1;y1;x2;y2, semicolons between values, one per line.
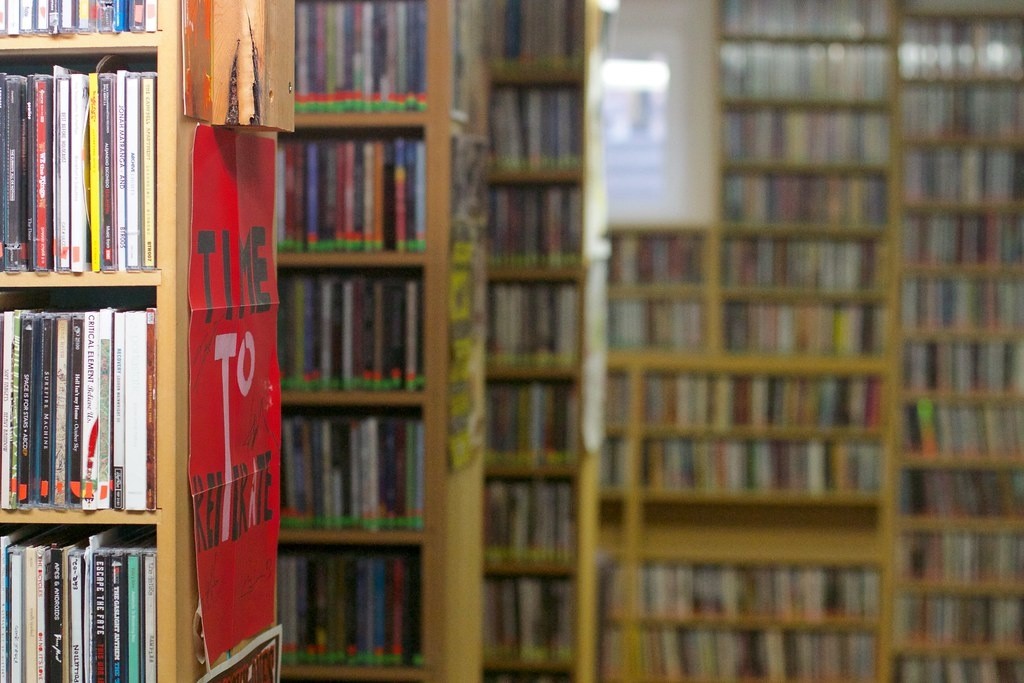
0;0;1024;683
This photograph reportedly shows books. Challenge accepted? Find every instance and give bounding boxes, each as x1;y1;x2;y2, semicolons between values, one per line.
277;0;427;668
0;0;158;683
482;0;581;663
599;0;1022;681
